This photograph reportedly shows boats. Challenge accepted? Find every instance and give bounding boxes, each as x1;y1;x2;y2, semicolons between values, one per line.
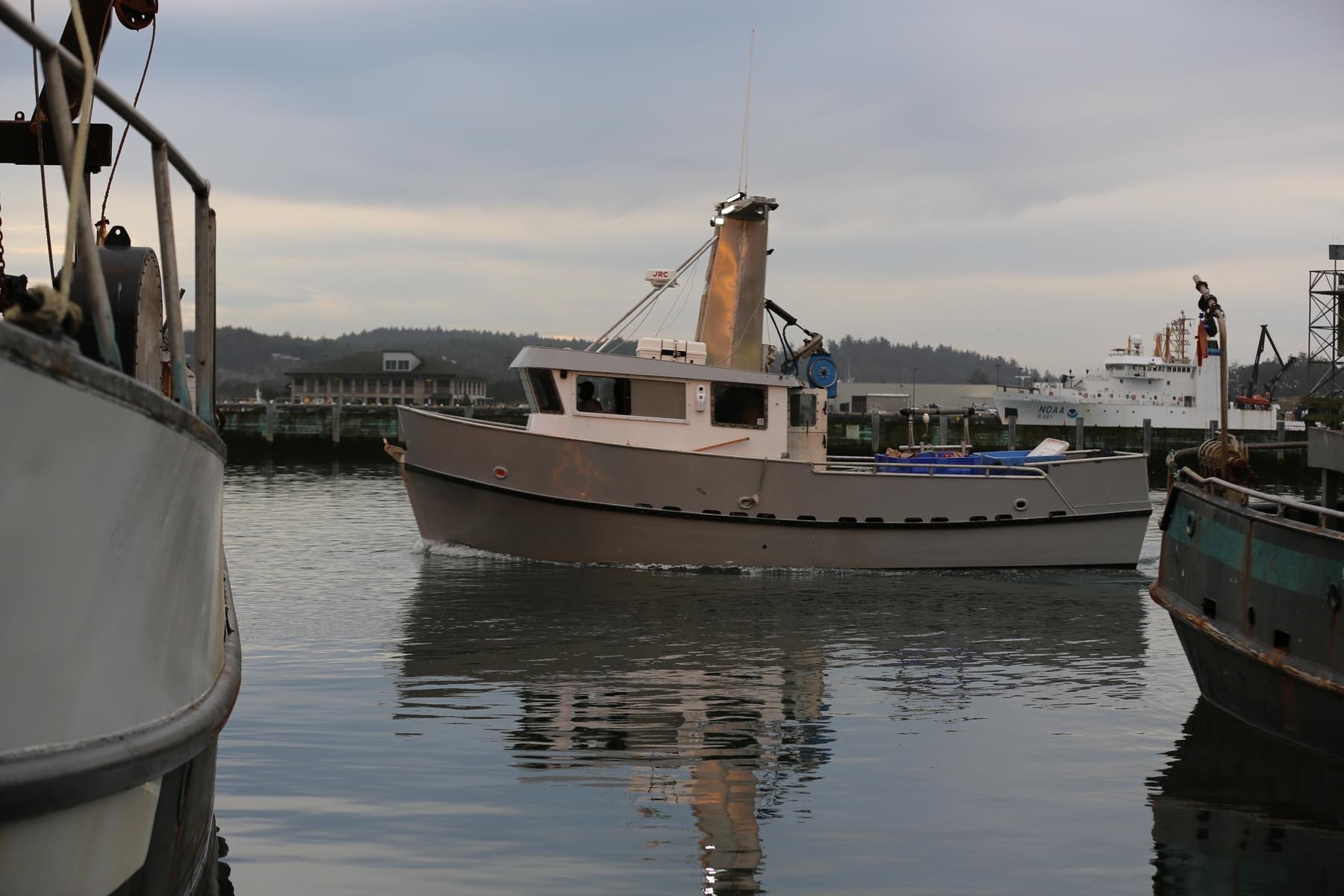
1146;426;1344;759
0;0;251;896
382;26;1153;572
993;309;1277;432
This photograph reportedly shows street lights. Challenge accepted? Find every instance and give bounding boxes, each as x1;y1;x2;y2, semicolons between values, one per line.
913;367;919;408
